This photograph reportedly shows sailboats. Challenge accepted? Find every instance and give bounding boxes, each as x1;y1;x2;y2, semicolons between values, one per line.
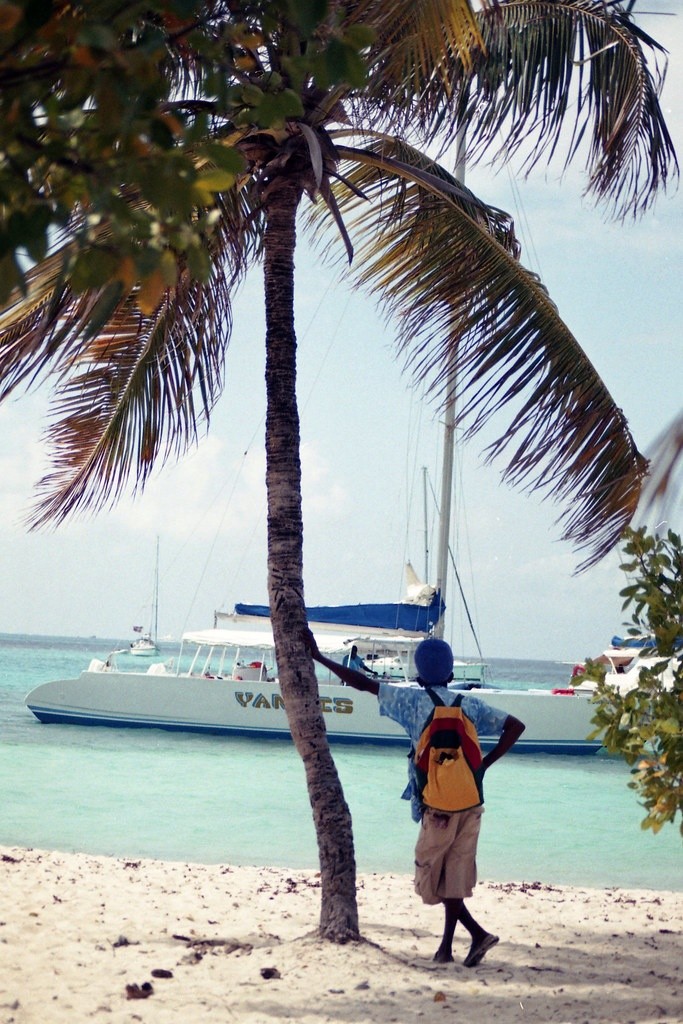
130;532;160;656
360;465;488;687
23;76;613;757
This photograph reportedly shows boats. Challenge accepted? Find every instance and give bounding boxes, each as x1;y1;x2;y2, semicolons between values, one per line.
527;635;680;699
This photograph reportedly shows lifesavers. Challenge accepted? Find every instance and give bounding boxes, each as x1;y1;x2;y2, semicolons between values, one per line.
571;664;586;677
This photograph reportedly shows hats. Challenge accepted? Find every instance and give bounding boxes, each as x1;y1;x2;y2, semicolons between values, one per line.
413;638;452;683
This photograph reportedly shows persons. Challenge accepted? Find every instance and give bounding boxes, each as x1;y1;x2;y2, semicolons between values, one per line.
306;630;526;968
342;645;378;686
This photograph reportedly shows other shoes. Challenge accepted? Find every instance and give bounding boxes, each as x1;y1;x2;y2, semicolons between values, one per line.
433;951;455;964
464;934;499;967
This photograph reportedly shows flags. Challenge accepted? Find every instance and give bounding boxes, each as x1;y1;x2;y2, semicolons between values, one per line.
133;626;143;633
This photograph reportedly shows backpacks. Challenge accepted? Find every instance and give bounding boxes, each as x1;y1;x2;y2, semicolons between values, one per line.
410;688;488;813
